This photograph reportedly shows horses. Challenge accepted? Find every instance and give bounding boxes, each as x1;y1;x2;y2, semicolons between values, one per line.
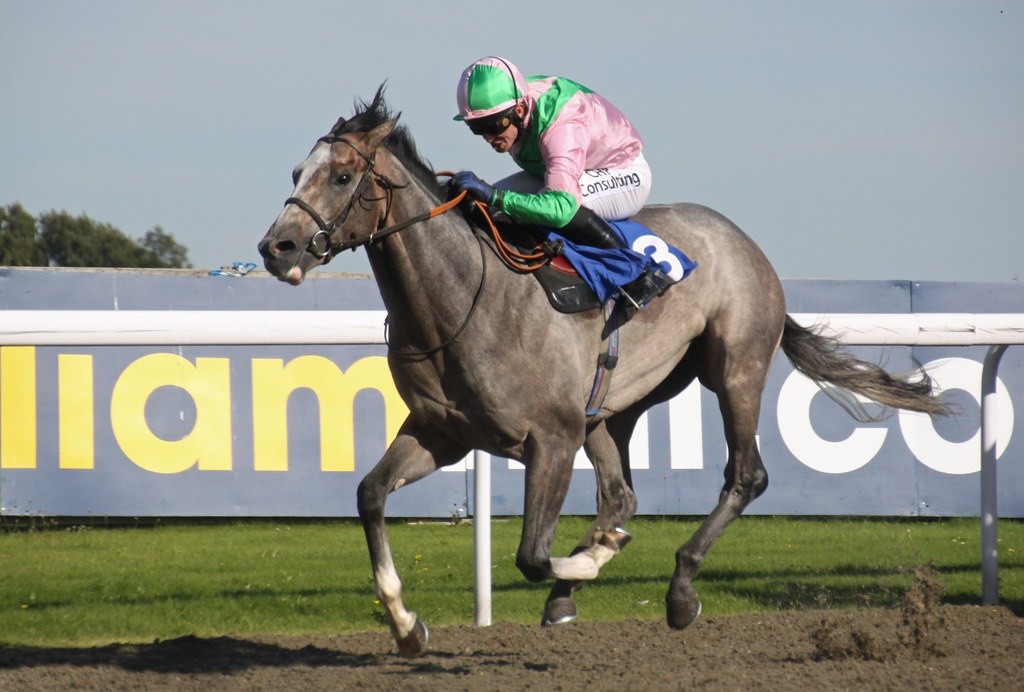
257;78;962;660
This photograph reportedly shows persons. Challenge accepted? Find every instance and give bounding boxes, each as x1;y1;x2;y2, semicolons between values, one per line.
447;57;676;318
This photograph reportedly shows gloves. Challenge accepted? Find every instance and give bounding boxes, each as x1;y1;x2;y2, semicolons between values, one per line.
450;170;494;203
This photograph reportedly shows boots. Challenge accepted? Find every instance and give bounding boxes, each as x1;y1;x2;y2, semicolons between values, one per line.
571;210;669;322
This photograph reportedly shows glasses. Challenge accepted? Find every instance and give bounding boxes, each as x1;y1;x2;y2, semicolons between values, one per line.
469;108;516;136
